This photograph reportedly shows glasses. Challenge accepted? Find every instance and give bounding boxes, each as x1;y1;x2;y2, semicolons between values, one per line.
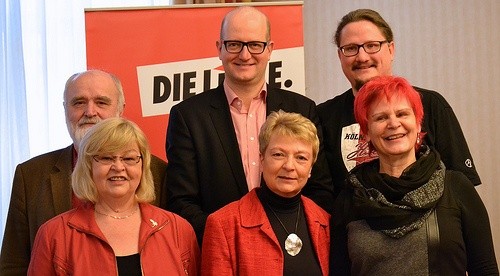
340;40;390;57
221;40;268;54
93;155;141;165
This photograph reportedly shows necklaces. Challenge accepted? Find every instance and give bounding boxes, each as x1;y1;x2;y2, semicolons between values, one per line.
95;210;140;219
270;202;302;256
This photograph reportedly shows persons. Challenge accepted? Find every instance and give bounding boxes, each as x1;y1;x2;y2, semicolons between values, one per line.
27;116;200;276
331;75;499;276
316;8;482;189
201;110;331;276
0;70;168;276
161;5;322;246
